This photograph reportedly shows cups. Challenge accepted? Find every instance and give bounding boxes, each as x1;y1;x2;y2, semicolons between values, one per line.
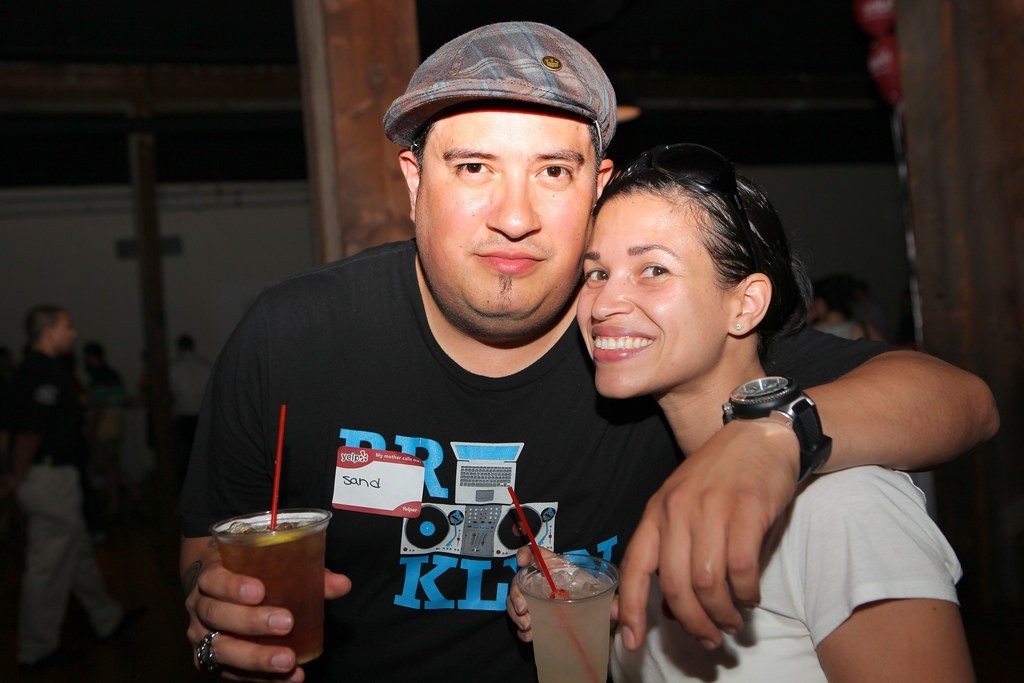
210;509;332;664
516;554;621;683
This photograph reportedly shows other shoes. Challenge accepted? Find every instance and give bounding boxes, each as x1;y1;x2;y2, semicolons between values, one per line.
96;604;143;644
18;644;84;672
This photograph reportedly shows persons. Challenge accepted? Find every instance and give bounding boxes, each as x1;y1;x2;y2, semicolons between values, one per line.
0;347;25;542
168;332;211;477
808;276;915;348
83;342;136;519
506;171;975;683
177;20;1000;683
0;304;146;676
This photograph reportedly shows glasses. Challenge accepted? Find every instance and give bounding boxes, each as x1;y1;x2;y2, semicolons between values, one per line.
592;144;760;273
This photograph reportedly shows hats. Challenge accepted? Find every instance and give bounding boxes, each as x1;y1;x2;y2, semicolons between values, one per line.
384;22;618;158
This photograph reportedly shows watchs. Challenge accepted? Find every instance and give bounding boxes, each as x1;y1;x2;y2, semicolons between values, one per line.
723;376;831;484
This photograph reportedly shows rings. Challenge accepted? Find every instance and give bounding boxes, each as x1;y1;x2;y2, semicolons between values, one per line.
197;631;218;670
